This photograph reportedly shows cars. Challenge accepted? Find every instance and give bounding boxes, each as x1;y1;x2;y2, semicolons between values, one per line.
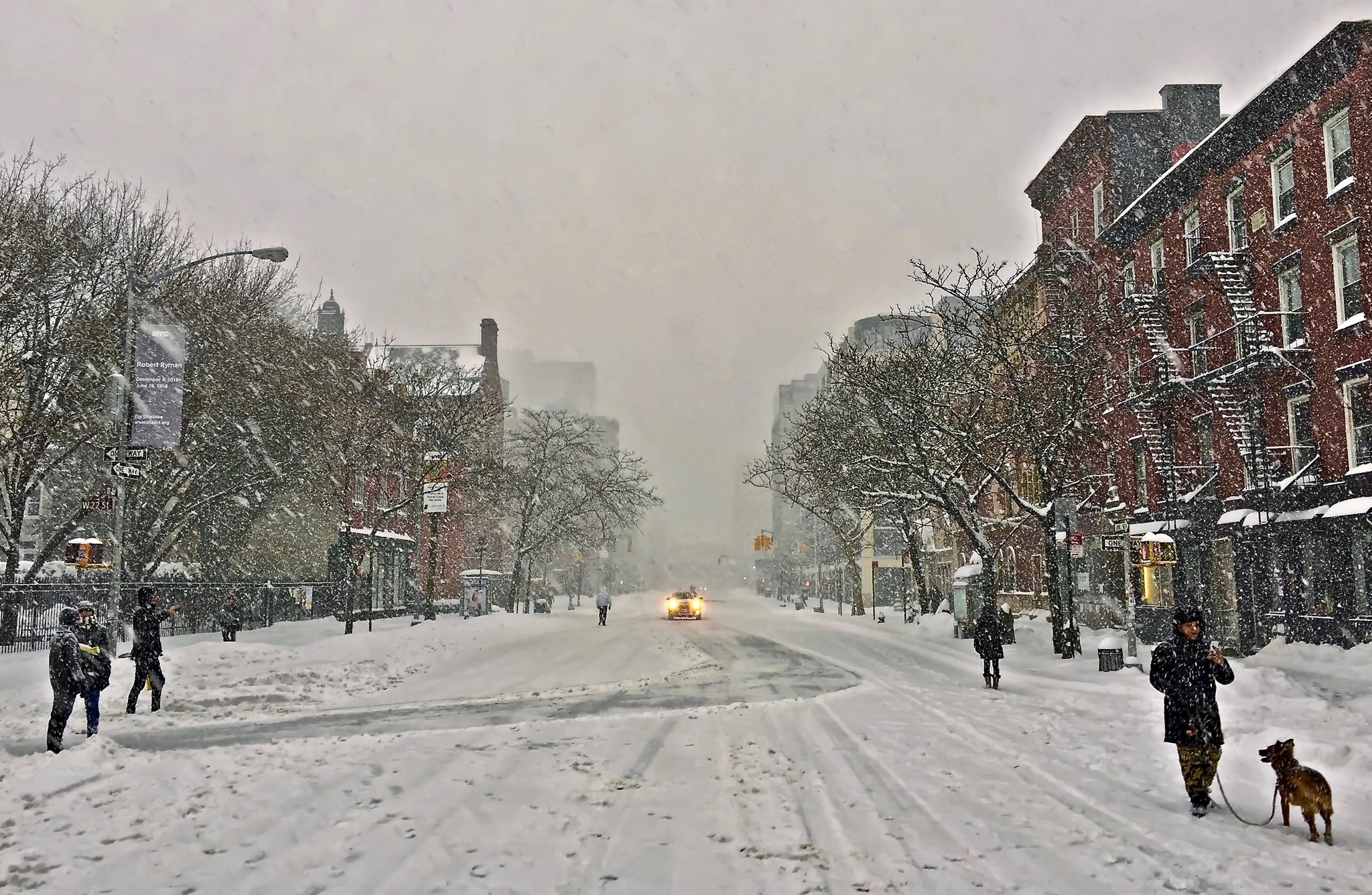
666;592;703;620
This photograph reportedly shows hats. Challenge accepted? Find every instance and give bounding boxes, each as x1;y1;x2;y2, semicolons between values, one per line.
59;608;79;625
77;600;94;614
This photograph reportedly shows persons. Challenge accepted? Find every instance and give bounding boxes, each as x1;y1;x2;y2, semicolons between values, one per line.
974;606;1004;690
1149;606;1235;818
689;585;695;592
124;585;178;715
927;585;943;615
46;607;83;755
547;593;554;608
218;596;240;642
595;586;612;626
465;591;482;617
798;593;807;606
75;600;111;738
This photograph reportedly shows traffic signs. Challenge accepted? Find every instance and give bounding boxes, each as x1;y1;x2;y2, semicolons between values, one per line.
111;460;144;481
1101;536;1142;553
103;444;148;462
1113;517;1139;534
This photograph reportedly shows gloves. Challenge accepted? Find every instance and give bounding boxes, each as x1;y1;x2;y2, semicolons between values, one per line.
79;678;90;698
975;647;980;654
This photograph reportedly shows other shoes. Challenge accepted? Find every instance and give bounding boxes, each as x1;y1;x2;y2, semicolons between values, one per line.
1190;795;1216;816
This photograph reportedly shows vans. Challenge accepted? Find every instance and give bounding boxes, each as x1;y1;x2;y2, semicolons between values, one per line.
756;577;775;595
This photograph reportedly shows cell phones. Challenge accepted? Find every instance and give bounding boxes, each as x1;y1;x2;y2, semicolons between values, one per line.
1210;641;1219;654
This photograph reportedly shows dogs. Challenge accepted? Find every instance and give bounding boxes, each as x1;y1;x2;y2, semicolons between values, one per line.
1258;738;1334;847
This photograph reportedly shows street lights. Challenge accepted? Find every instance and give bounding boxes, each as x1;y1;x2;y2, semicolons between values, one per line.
103;245;293;656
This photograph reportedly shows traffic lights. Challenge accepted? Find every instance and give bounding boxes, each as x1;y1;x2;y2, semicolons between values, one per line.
1155;541;1180;565
88;544;107;566
805;581;812;588
65;542;83;565
1140;542;1155;564
754;535;772;551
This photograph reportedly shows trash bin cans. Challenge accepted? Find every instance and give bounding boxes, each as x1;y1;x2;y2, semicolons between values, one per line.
1098;648;1123;673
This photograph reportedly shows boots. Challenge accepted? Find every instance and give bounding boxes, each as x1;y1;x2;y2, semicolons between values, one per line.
983;673;992;688
991;673;1001;689
